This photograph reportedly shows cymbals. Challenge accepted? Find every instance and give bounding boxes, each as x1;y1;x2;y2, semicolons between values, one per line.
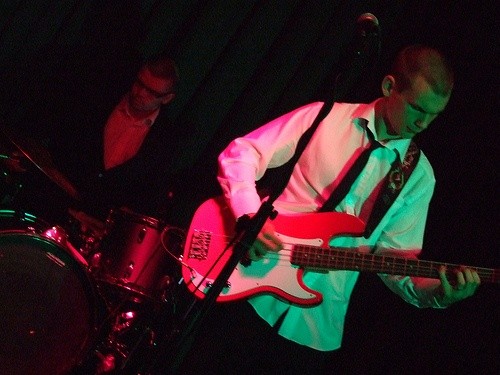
0;121;84;204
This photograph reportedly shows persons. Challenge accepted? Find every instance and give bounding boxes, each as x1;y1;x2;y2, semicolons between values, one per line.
37;52;189;222
181;45;481;375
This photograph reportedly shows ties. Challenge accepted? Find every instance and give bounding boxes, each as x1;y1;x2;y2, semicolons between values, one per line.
318;122;384;212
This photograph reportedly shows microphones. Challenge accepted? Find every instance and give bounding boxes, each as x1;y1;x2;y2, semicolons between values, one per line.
355;12;380;63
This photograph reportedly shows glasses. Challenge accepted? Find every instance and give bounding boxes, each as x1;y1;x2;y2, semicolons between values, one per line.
136;79;176;99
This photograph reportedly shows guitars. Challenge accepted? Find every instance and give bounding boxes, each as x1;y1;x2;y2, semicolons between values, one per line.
181;196;500;308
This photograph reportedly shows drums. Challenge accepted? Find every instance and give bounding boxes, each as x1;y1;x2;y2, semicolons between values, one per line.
63;207;110;260
0;209;99;375
94;204;171;304
